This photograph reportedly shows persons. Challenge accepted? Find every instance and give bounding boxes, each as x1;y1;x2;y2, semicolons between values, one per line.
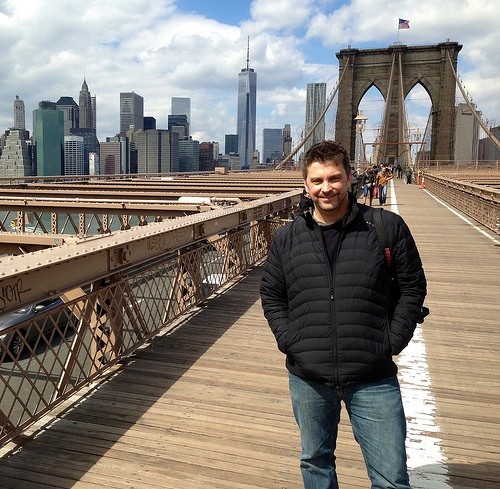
260;141;429;489
298;189;314;214
350;160;413;207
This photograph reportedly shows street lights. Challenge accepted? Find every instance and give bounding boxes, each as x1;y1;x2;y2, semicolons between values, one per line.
414;128;421;176
354;110;368;174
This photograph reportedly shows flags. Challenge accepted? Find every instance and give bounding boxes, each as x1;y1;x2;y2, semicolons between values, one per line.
399;19;410;29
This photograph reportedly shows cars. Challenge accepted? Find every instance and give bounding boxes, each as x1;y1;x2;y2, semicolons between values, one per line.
0;287;90;359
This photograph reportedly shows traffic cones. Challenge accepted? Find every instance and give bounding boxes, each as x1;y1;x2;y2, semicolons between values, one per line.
419;176;427;189
415;176;421;185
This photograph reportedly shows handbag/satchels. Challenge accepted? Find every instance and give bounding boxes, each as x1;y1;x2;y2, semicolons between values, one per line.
375;187;379;199
